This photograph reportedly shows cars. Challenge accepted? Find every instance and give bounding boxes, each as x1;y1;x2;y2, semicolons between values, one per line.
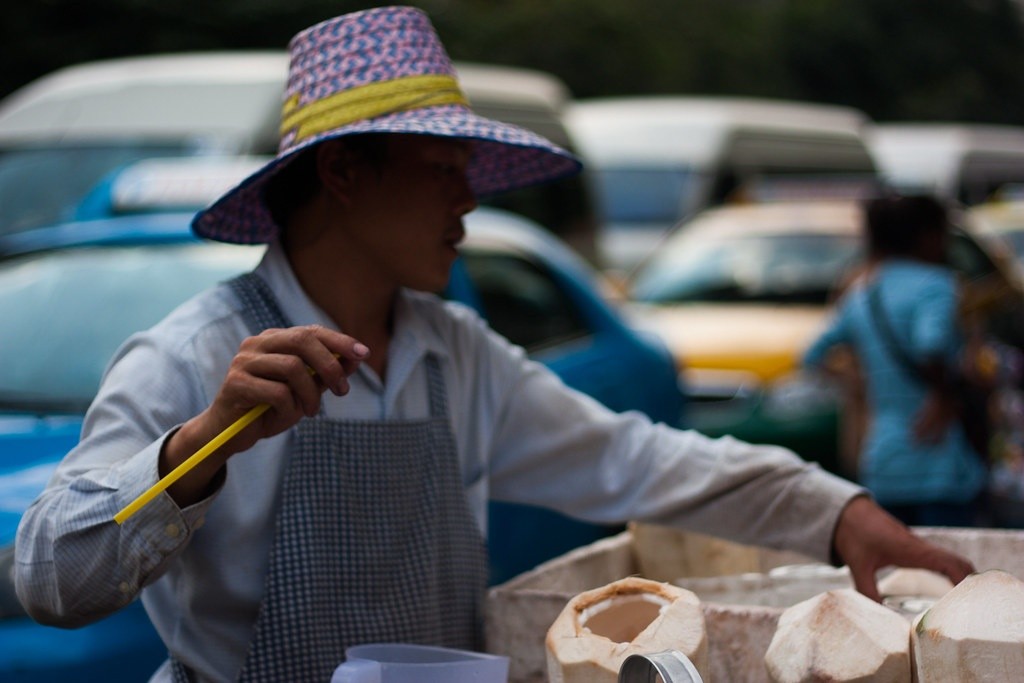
0;155;684;683
867;116;1024;300
613;201;869;470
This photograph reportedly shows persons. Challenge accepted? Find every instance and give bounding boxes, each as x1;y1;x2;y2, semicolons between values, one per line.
798;185;993;527
14;7;980;682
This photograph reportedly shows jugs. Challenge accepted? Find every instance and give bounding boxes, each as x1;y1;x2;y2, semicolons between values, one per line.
331;644;510;683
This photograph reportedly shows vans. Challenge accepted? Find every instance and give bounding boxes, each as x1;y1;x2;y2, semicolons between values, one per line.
0;53;603;273
560;94;888;287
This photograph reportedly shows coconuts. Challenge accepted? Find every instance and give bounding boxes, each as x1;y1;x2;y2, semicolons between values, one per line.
544;577;709;683
765;587;912;683
912;570;1024;683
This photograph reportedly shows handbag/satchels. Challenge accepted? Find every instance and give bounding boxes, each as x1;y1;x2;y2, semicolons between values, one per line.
939;371;996;470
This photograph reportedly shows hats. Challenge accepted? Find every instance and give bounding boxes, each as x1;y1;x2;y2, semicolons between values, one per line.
191;7;583;246
